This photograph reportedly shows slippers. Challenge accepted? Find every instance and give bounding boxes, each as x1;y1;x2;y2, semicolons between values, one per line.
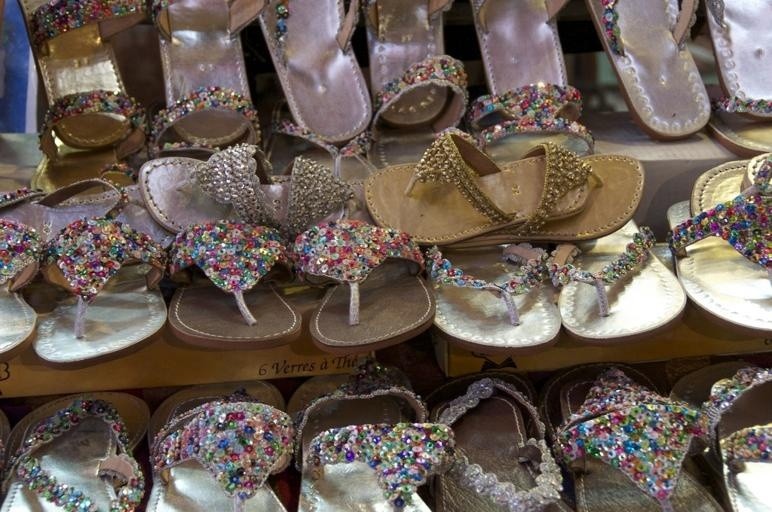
427;370;569;511
541;363;723;511
285;365;458;510
2;392;152;512
148;381;295;512
3;3;771;371
666;357;770;512
